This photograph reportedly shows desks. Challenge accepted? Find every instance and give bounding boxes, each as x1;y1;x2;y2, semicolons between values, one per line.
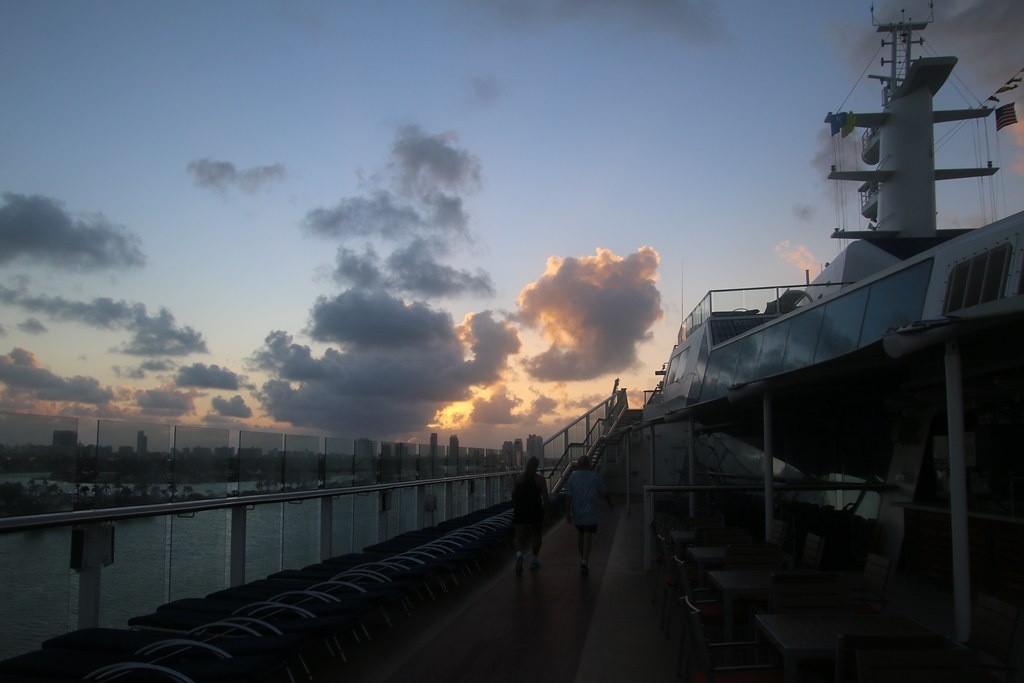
685;547;794;588
753;614;952;683
707;570;832;666
668;530;748;560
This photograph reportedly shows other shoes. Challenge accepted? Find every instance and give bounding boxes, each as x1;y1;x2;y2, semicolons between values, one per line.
528;562;541;569
516;555;523;575
580;565;591;572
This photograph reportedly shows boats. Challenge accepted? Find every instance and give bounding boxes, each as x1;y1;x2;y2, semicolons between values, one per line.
0;0;1024;683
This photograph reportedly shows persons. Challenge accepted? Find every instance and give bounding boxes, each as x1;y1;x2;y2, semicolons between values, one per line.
512;456;550;575
565;456;614;577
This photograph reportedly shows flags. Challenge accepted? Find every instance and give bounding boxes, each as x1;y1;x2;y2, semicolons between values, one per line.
987;67;1024;103
832;111;847;135
841;112;856;137
995;102;1018;131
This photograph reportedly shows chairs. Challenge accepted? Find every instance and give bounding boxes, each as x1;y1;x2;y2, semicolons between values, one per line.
653;515;981;683
0;493;564;683
